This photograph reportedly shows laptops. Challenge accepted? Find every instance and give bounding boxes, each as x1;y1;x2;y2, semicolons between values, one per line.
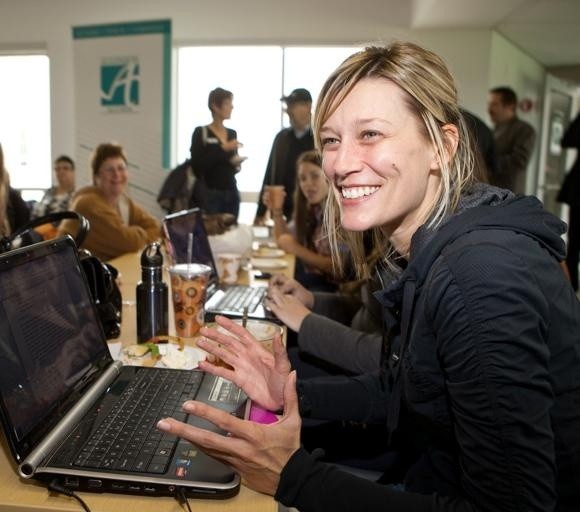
0;236;249;499
164;208;280;321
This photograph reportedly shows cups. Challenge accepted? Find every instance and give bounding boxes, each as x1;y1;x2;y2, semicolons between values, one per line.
218;252;241;285
169;262;211;338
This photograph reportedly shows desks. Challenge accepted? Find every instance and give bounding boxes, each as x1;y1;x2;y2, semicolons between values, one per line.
0;217;297;512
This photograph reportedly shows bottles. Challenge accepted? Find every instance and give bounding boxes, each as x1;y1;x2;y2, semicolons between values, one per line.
136;242;168;344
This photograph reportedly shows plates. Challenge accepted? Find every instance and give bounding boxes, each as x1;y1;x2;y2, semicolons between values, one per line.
252;258;290;269
253;249;285;258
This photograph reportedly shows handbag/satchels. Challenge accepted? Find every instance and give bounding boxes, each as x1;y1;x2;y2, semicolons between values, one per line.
0;211;123;340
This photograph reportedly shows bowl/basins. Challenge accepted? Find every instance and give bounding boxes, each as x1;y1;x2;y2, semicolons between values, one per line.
206;320;284;370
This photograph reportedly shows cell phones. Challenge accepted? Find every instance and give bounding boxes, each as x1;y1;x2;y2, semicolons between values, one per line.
253;271;272;278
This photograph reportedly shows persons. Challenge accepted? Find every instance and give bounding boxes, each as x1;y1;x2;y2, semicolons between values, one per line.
263;152;351;293
0;142;30;254
190;87;246;227
555;112;580;293
158;43;580;512
31;155;78;227
488;87;535;196
262;107;499;378
57;144;159;261
253;88;314;225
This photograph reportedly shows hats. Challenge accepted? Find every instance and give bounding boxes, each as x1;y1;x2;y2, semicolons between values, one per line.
281;89;312;102
